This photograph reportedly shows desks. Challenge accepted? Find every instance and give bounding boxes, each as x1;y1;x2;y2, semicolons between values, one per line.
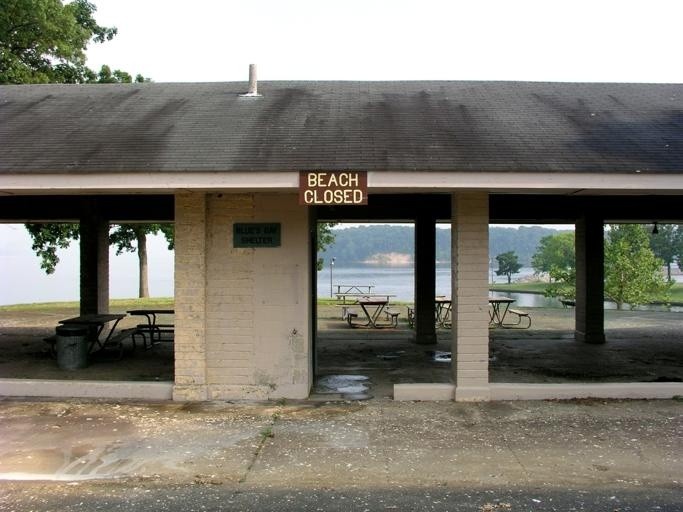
57;312;123;358
127;307;174;344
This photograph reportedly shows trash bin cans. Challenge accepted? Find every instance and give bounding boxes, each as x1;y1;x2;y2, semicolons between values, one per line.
55;324;88;372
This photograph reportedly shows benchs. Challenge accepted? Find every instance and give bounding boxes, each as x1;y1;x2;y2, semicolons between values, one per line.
332;287;533;330
44;330;54;358
107;328;141;359
139;323;174;344
